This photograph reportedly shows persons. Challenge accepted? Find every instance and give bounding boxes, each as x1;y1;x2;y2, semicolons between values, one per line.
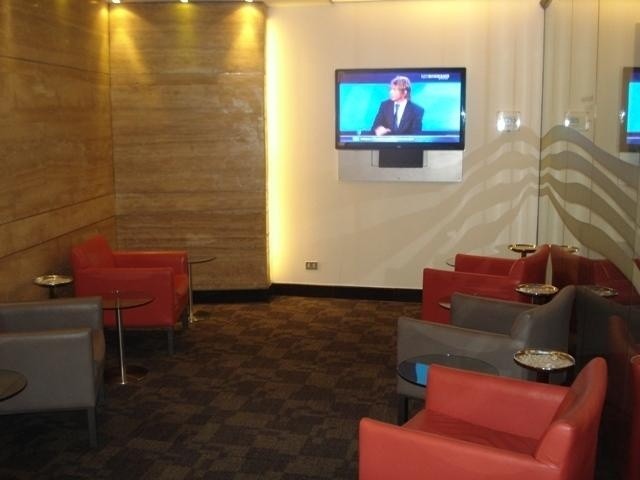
371;75;424;136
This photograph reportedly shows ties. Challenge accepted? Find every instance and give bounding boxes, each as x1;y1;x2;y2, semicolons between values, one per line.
393;104;400;129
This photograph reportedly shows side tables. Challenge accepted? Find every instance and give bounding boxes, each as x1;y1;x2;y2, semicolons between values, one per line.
102;290;156;385
187;255;217;322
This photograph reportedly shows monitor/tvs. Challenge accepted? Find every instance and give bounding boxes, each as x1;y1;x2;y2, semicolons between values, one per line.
335;67;466;150
618;67;640;152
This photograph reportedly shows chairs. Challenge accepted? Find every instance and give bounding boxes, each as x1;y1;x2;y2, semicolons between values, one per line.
70;234;190;356
0;296;107;446
